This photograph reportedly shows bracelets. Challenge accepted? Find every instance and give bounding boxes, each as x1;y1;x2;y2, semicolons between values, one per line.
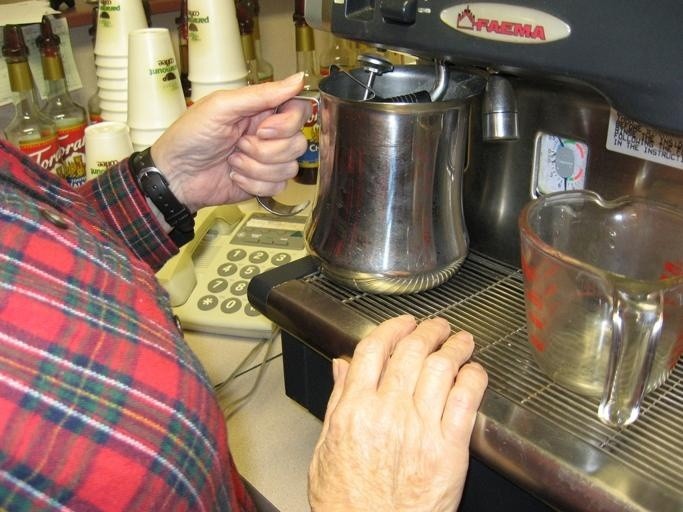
126;147;198;251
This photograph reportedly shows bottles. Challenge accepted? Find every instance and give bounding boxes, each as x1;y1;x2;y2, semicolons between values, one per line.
235;1;361;184
1;16;91;188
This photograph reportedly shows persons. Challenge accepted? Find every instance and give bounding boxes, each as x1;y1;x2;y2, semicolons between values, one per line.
1;70;490;512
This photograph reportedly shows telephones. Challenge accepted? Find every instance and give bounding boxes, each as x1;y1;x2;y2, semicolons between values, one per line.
152;204;309;340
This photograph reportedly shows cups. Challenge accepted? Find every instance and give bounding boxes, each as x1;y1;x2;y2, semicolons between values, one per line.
257;65;486;296
519;189;683;428
85;0;251;189
